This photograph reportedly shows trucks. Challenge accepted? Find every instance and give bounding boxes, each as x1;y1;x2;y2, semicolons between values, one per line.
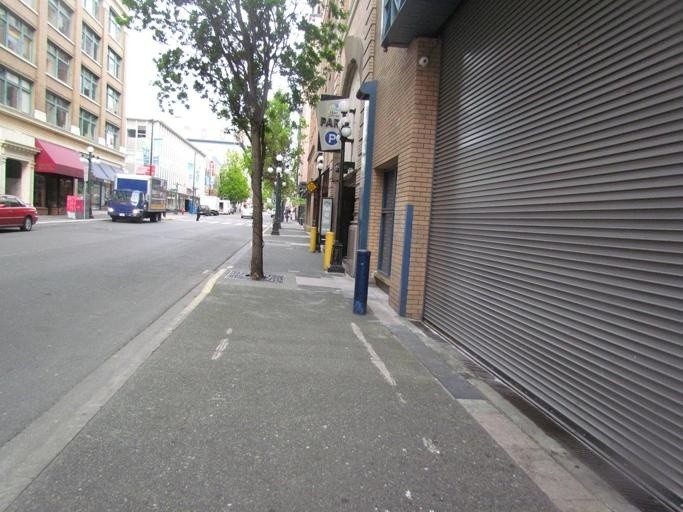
106;173;167;224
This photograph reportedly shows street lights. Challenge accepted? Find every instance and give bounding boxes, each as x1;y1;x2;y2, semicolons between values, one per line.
314;155;325;253
86;145;94;219
266;154;290;235
326;98;353;274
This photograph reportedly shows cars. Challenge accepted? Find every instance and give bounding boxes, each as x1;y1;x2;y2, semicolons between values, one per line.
196;196;277;218
1;195;38;232
